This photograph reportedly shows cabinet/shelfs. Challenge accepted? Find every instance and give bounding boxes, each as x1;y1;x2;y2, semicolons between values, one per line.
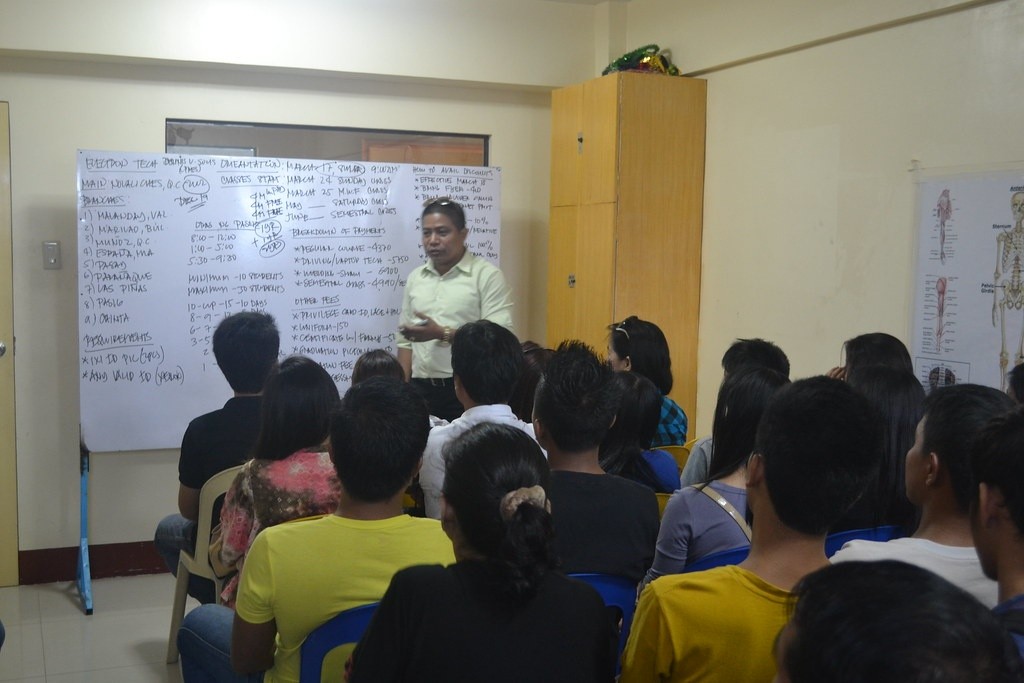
549;71;707;447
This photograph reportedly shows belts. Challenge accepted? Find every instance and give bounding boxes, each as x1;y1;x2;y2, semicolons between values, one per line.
411;378;454;386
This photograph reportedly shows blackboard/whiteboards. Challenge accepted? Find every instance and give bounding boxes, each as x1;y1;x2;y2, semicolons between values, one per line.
76;148;500;453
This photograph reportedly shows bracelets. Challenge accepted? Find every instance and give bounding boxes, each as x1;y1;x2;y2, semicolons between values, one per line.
443;327;450;344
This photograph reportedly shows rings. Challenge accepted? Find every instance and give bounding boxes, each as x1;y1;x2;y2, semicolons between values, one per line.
409;336;416;342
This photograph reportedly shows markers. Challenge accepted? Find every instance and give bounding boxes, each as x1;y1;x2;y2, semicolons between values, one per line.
399;319;427;335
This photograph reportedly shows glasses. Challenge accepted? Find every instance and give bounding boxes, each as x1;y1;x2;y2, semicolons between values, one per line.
614;313;638;345
422;197;464;225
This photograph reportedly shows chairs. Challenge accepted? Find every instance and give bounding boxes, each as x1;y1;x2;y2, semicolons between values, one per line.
571;573;638;660
300;603;380;682
167;461;249;665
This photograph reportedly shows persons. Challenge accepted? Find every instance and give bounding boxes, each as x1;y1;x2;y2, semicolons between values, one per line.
177;316;688;683
397;201;516;424
616;331;1024;683
350;347;406;388
154;309;280;607
219;355;340;603
